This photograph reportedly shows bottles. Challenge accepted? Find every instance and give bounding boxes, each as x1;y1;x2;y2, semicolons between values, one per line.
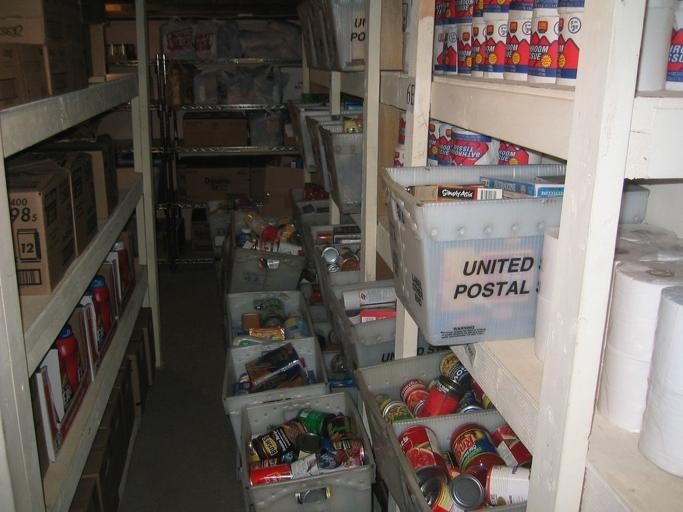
236;228;253;250
54;323;85;394
111;241;131;287
211;228;226;258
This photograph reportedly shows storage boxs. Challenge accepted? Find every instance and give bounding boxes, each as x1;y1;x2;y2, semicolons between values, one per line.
67;321;156;512
300;0;403;69
3;0;89;112
8;135;119;299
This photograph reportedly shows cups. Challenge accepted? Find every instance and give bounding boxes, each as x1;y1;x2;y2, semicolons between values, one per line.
273;239;279;253
277;240;303;254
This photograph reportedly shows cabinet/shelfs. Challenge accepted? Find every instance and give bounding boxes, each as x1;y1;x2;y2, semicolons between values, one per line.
0;0;160;512
293;0;683;511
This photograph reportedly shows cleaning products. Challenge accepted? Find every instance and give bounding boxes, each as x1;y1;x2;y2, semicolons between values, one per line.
55;324;86;402
86;291;106;352
59;360;76;412
114;240;131;293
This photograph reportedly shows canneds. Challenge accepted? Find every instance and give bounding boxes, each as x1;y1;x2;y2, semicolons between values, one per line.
320;246;340;266
375;353;493;422
237;403;365;486
397;422;532;511
